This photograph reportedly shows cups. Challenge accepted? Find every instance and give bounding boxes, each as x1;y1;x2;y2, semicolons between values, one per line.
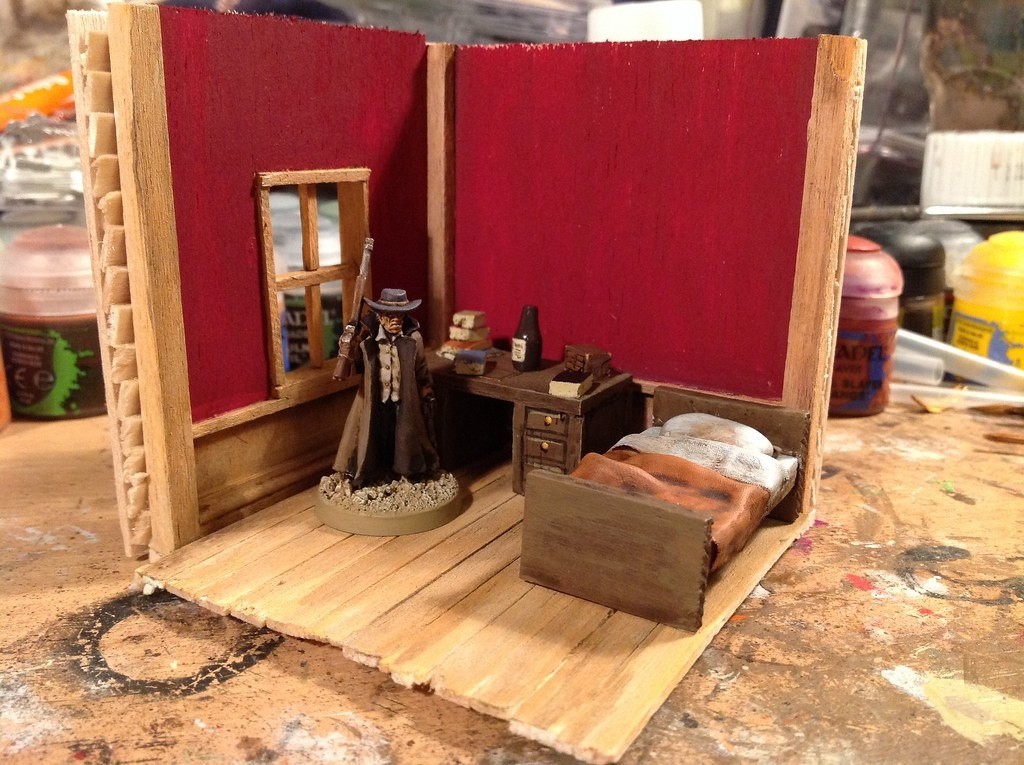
0;205;106;422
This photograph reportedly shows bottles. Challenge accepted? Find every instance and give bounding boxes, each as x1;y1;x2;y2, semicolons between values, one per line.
828;217;1024;415
510;304;542;370
